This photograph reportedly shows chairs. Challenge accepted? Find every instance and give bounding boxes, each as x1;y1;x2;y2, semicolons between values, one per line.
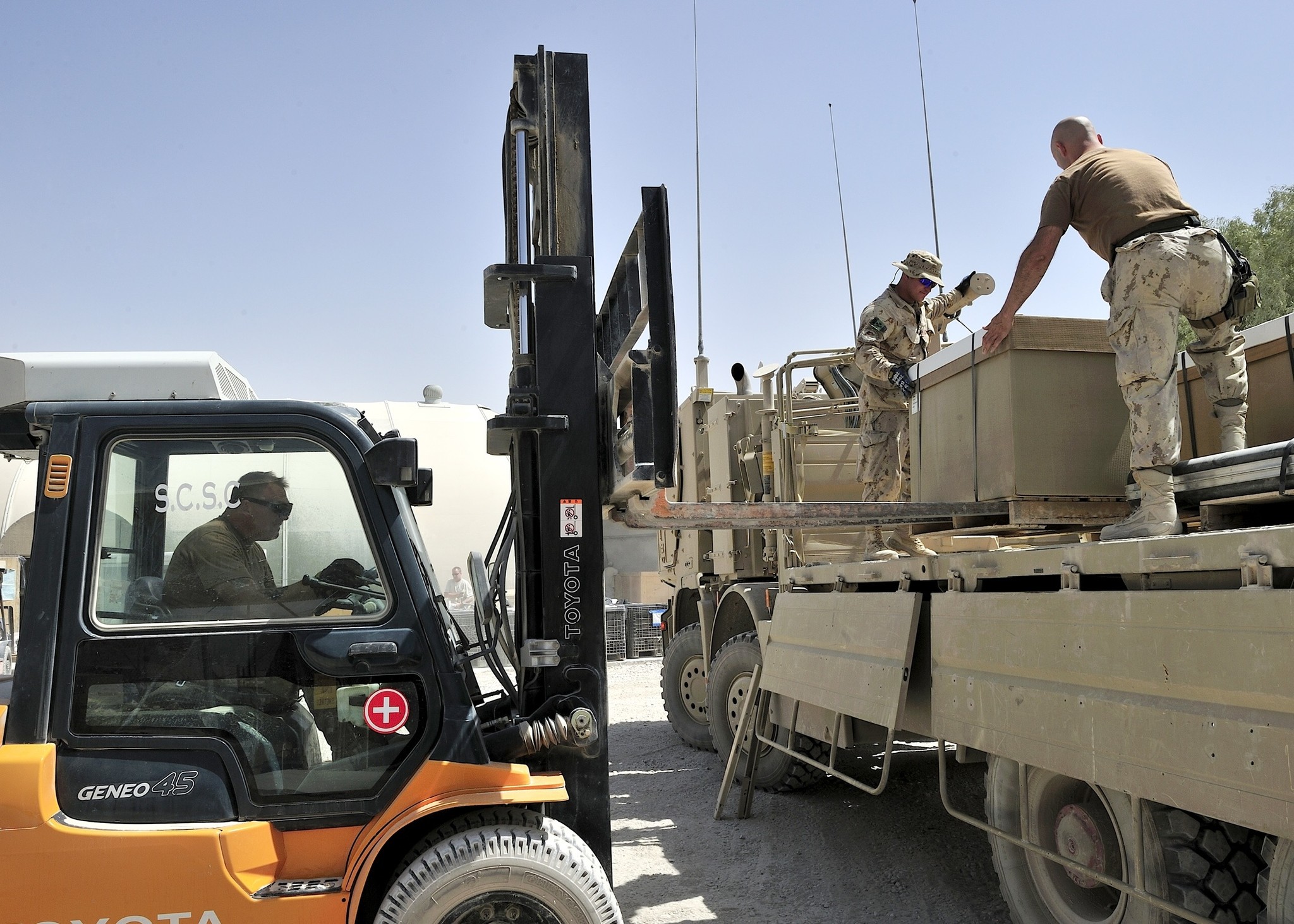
122;576;300;720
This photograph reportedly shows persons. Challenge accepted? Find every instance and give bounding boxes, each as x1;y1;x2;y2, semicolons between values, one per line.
980;116;1248;540
444;567;475;611
162;472;389;770
854;250;976;561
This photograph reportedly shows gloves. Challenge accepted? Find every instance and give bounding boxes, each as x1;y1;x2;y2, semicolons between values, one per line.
955;271;976;298
887;363;917;400
311;558;366;599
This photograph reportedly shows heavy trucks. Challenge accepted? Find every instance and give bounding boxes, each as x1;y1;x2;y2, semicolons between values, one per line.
655;268;1294;923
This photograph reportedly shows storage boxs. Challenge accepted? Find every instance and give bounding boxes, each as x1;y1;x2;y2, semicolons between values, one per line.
907;315;1134;503
1175;311;1294;461
605;603;669;661
448;607;515;667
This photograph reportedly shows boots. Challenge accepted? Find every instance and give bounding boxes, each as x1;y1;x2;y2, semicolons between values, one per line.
1099;469;1182;539
1211;402;1248;453
886;524;937;556
863;525;899;561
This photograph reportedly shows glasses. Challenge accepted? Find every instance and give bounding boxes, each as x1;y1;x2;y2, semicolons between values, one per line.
452;573;460;575
918;278;938;288
239;497;294;521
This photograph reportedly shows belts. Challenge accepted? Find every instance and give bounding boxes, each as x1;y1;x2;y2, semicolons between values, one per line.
1110;215;1201;266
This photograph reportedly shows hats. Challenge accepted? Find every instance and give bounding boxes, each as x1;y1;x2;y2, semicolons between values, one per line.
891;250;945;286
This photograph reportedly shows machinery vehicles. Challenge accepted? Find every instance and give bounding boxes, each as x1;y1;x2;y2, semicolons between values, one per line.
0;345;517;679
0;45;683;924
